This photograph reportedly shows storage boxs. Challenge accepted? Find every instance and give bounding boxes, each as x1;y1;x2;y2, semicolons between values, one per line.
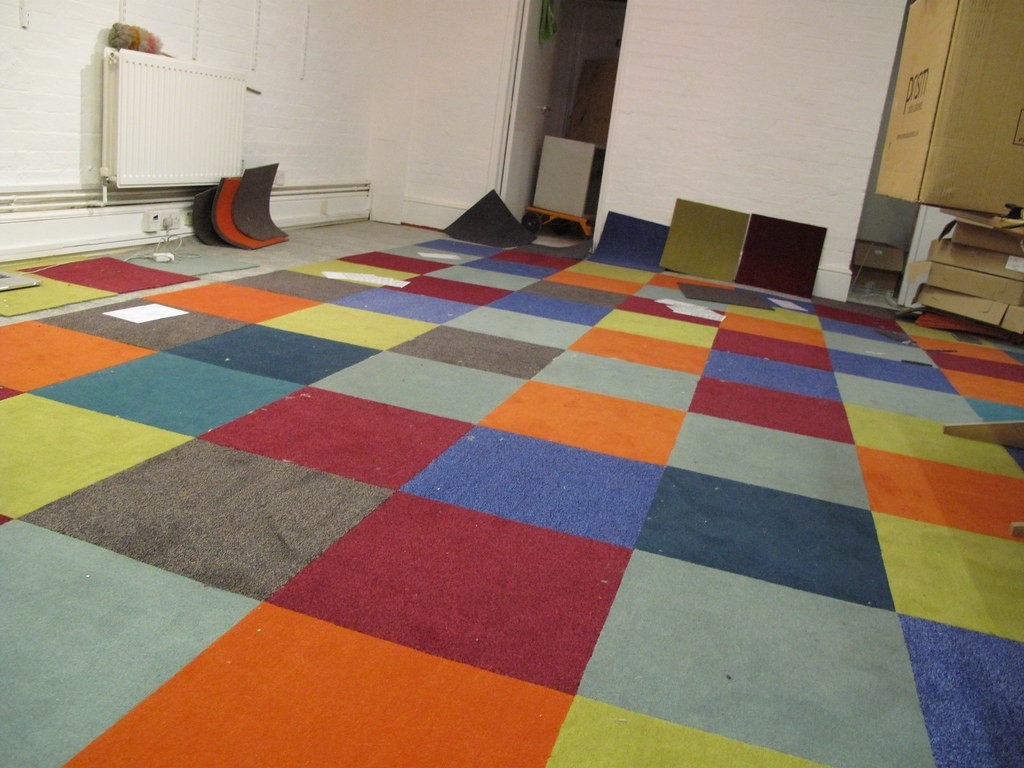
850;0;1024;337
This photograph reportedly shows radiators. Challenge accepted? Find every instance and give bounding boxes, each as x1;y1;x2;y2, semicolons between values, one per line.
101;47;246;188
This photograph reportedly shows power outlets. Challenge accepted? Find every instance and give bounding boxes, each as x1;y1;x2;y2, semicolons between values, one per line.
160;212;182;230
